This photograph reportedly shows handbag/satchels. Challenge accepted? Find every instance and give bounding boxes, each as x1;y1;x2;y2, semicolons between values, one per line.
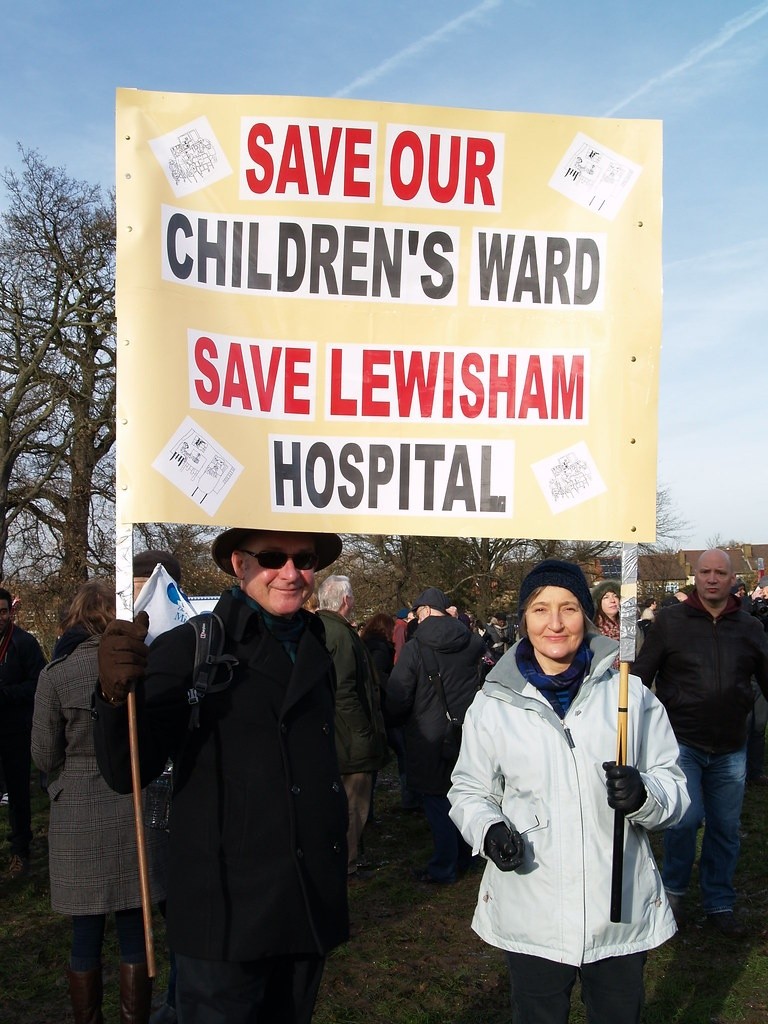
437;718;462;762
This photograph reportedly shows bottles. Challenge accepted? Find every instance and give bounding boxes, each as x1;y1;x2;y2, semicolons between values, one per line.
143;757;175;831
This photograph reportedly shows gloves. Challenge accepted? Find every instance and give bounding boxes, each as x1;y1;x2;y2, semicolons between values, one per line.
485;823;524;871
97;611;149;705
603;761;647;813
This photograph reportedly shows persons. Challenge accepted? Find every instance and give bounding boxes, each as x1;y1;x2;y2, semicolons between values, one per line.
589;580;644;675
660;584;694;608
313;574;388;881
732;580;768;787
0;588;49;883
386;587;491;886
356;607;420;816
90;527;351;1024
32;584;171;1024
445;605;514;691
640;598;657;623
134;562;198;640
448;556;691;1024
630;548;768;939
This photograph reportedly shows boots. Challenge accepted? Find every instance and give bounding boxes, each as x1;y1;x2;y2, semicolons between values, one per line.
118;961;151;1024
68;964;103;1024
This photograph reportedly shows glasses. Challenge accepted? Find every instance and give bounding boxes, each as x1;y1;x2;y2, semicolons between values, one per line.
413;607;425;620
239;548;318;570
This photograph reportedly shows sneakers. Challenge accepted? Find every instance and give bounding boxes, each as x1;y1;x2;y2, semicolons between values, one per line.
750;774;768;785
708;911;746;939
7;855;27;881
667;892;680;913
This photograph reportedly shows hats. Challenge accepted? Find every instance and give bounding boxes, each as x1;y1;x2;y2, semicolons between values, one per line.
592;581;621;611
410;587;445;611
211;527;342;576
396;609;409;619
518;558;594;620
133;550;180;583
759;576;768;587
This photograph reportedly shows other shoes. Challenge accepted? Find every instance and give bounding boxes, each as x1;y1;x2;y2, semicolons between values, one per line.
416;870;440;882
348;869;372;883
392;806;420;813
